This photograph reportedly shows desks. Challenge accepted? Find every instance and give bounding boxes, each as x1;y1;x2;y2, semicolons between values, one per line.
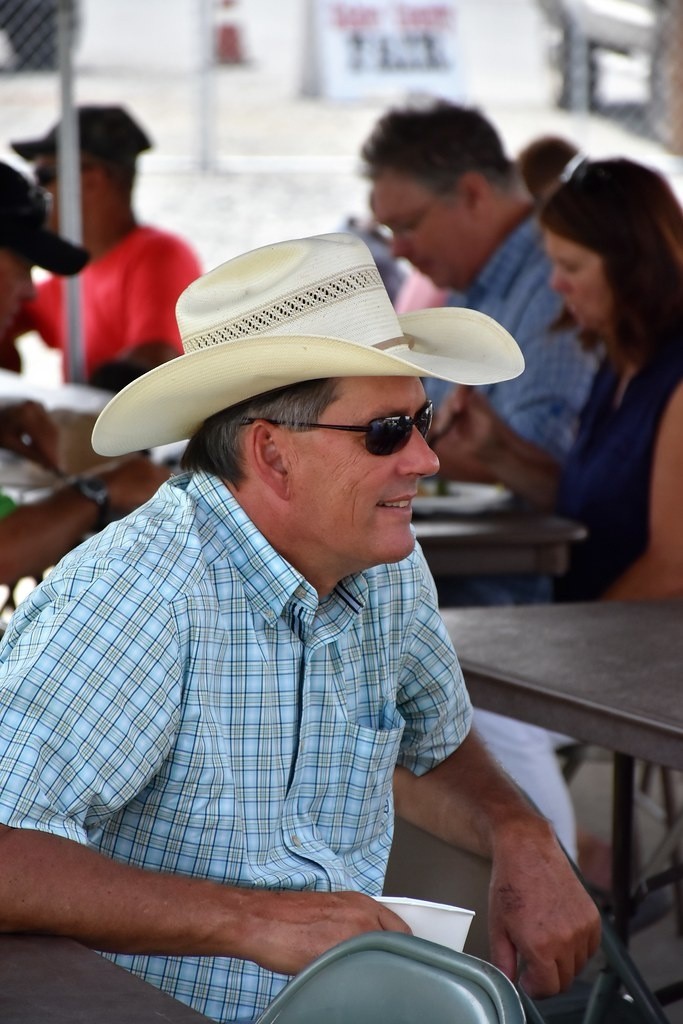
1;924;221;1023
50;411;587;581
438;595;683;1007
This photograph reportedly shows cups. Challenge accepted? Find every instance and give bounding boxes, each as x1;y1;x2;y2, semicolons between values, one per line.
22;349;67;393
371;895;478;955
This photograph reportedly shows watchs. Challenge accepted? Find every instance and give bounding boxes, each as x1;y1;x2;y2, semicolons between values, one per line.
71;472;113;533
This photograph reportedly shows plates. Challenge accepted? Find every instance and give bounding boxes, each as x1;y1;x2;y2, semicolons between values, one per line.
408;476;511;514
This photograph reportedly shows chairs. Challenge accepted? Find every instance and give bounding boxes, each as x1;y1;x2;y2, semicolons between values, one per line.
243;815;527;1024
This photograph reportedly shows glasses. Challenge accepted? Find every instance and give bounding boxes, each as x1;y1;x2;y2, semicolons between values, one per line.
33;165;94;187
560;151;621;225
237;400;434;456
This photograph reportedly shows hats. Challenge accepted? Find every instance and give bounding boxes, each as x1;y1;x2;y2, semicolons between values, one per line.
91;233;524;457
12;106;150;165
0;163;89;276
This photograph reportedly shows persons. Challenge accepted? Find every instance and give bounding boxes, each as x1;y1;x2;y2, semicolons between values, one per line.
1;231;602;1024
0;98;683;920
